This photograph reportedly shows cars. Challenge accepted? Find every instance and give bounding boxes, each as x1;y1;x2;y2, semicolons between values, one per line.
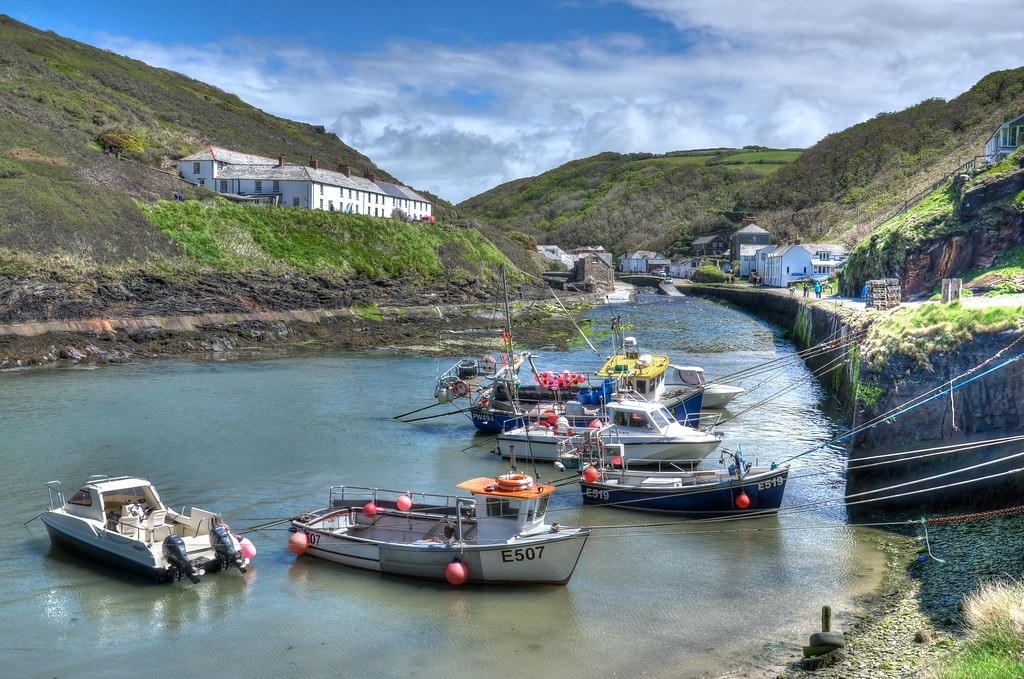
652;270;667;277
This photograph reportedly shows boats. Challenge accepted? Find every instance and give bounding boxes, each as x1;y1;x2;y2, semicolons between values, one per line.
554;424;791;520
435;263;746;467
287;425;592;586
41;475;249;584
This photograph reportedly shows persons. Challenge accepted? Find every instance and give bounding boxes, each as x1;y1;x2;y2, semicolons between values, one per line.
753;276;762;287
815;282;822;299
802;284;808;297
726;276;735;283
860;281;871;303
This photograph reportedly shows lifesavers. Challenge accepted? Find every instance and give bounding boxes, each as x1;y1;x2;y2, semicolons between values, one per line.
552;422;573;436
452;380;469;397
495;473;534;492
480;355;497;372
